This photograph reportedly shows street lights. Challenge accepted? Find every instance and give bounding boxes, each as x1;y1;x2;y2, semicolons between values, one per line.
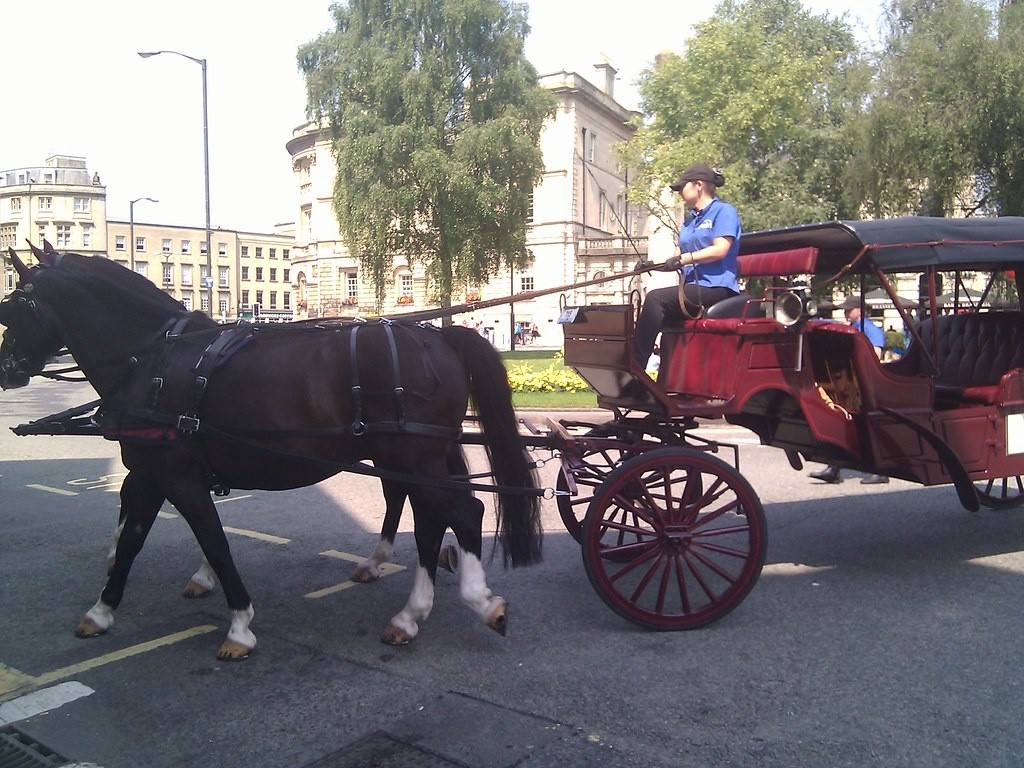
509;169;568;349
130;197;157;269
138;49;213;319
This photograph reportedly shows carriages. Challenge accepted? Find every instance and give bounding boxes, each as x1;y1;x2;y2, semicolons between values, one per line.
1;215;1023;663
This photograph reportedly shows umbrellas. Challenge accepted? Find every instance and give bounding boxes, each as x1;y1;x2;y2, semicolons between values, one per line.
817;288;920;331
922;288;1009;313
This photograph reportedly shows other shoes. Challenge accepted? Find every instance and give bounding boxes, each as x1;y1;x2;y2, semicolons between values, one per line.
810;467;838;483
860;471;890;485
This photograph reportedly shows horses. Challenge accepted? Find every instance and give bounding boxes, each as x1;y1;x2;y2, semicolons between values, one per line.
2;238;546;664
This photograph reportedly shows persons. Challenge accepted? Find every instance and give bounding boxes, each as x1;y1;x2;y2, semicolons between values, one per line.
633;162;741;370
467;317;488;339
806;297;890;484
515;321;541;344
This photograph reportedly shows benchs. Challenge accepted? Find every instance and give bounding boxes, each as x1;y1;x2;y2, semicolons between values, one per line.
878;310;1023;406
660;246;820;335
804;320;934;408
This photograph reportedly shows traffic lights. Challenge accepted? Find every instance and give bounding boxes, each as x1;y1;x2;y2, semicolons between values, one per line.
253;302;261;318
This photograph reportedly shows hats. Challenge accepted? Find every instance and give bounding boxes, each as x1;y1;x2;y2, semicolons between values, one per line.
837;296;867;310
669;164;719;192
